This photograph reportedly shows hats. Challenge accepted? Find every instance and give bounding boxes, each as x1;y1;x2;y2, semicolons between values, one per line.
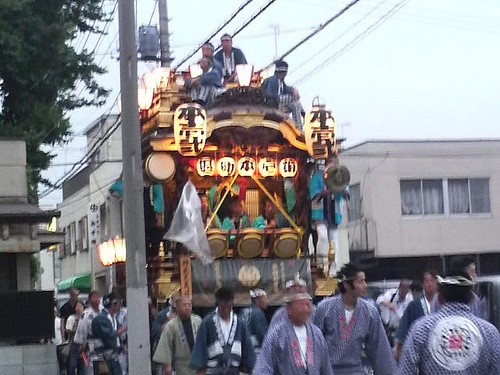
214;287;233;302
249;287;266;298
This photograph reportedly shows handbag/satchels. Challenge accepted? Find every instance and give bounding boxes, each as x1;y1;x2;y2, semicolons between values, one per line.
56;342;70;365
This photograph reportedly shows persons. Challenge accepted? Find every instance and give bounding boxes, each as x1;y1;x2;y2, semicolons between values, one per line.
237;287;268;355
91;294;125;375
215;34;248;82
222;196;249;240
153;297;203;375
53;288;104;375
188;288;256;375
376;279;423;345
269;277;316;332
261;61;304;131
198;194;221;228
185;42;226;107
118;291;180;375
398;268;500;375
254;197;292;245
431;257;480;319
393;271;436;362
254;291;336;375
313;264;398;375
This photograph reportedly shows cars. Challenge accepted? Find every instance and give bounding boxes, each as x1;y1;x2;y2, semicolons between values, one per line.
363;275;500;349
55;292;91;318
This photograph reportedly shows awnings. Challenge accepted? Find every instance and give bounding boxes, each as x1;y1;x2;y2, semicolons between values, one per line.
58;274;91;294
